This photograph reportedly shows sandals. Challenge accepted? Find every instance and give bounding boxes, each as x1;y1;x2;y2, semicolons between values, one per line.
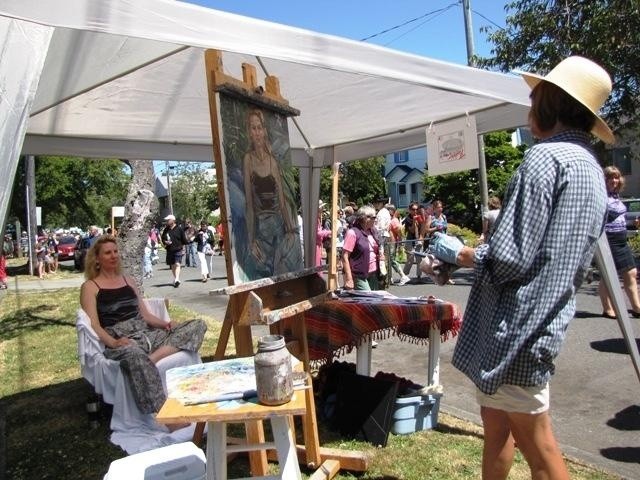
603;311;617;319
630;311;639;316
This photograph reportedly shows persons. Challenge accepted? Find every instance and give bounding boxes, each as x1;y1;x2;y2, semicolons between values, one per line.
401;200;434;283
193;217;215;282
184;218;199;268
161;215;187;288
382;203;411;286
339;202;358;223
315;199;332;278
46;233;59;274
151;221;162;265
371;194;394;287
35;235;47;279
81;234;207;431
19;230;29;260
342;201;358;227
341;205;385;291
335;205;347;290
0;246;9;291
85;225;101;247
599;165;640;319
431;54;615;480
480;195;502;234
323;205;345;275
216;219;231;256
142;230;153;279
207;222;216;237
241;109;301;280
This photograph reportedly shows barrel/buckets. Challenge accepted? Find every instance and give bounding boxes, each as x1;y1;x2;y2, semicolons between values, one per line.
254;335;294;406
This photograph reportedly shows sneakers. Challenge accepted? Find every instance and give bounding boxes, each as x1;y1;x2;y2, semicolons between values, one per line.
396;276;410;285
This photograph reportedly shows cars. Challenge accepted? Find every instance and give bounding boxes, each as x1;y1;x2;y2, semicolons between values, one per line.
73;238;98;270
54;236;78;260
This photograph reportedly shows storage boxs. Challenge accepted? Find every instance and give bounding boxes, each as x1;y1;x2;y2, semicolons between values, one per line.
390;388;443;436
103;441;207;479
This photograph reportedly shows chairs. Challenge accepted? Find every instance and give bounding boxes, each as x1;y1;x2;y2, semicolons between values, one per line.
76;298;200;417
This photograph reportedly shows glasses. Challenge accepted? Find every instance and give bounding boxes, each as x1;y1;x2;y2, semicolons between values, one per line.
410;208;419;211
367;215;377;220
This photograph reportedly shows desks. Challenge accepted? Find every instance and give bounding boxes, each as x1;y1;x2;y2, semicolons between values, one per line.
273;289;457;432
157;361;307;480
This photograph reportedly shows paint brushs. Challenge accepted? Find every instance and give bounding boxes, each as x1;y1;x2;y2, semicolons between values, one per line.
394;234;468;242
402;249;439;259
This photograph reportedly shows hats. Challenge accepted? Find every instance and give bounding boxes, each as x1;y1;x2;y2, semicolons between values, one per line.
318;198;327;208
164;214;176;221
510;55;616;145
